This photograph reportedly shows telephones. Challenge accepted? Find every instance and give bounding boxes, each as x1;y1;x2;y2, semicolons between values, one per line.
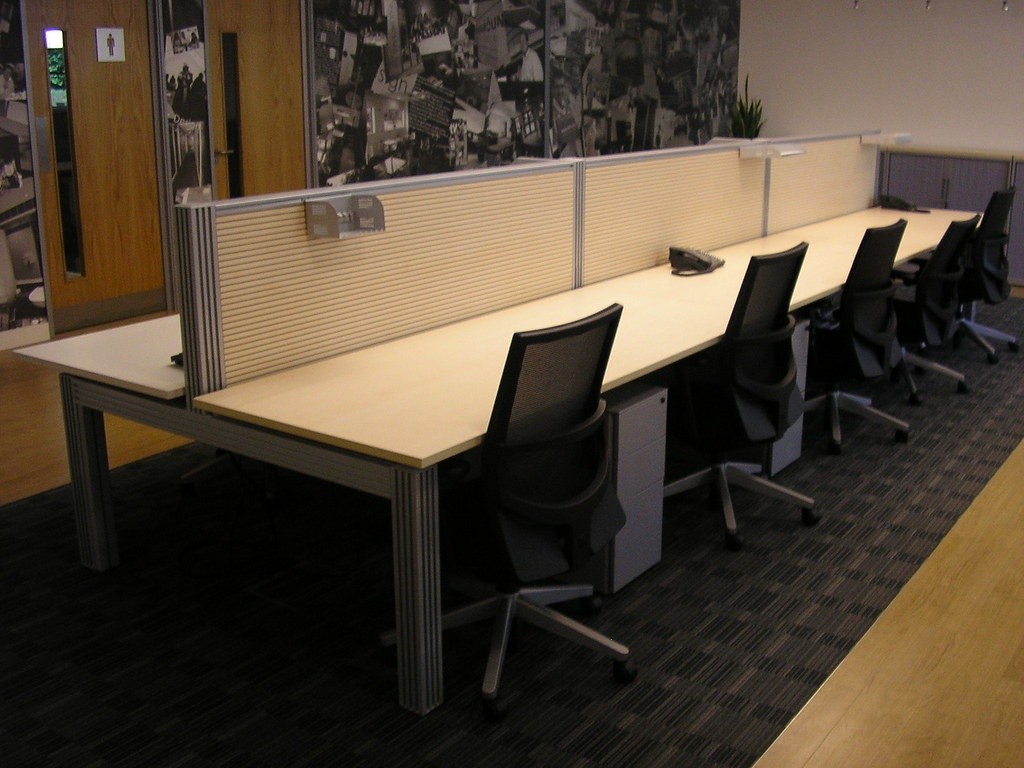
879;194;915;211
668;244;726;274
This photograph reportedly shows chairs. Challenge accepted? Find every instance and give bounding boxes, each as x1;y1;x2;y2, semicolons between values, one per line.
377;183;1016;710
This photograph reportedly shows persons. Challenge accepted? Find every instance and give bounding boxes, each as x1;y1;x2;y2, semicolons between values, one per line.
411;11;446;42
564;31;588;95
623;23;675;111
552;70;575;122
172;31;198;54
657;33;692;86
0;63;16;99
519;31;543;82
3;152;18;187
166;65;207;121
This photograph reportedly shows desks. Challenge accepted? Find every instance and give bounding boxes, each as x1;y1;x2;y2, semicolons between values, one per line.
11;127;992;715
